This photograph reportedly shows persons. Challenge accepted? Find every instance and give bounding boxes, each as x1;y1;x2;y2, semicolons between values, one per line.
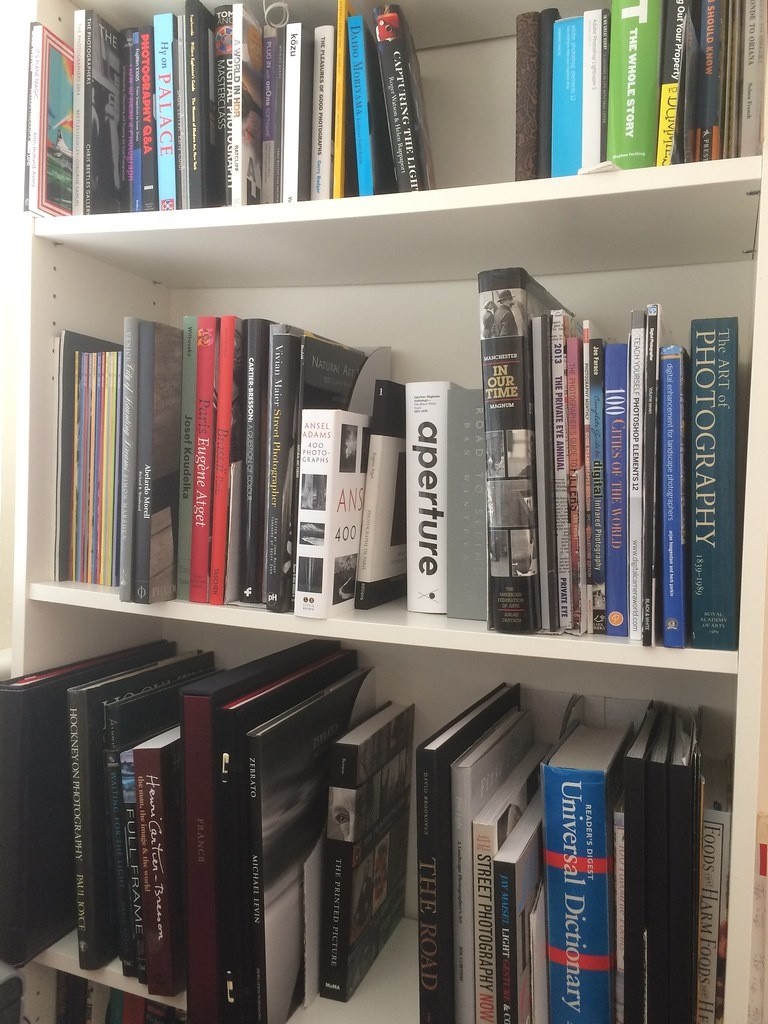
355;860;372;920
494;290;518;336
332;787;354;842
483;301;494;338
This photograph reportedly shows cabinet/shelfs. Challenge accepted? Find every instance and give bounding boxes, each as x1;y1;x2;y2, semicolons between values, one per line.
7;0;768;1023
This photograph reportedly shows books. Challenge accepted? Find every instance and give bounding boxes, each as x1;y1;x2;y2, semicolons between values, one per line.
54;267;741;652
23;0;767;216
1;638;731;1024
